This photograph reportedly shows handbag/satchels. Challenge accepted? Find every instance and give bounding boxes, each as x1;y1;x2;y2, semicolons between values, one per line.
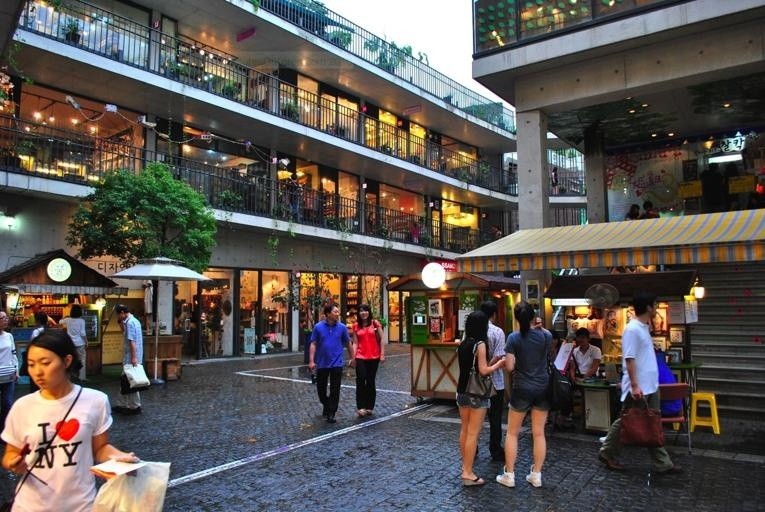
123;363;151;389
91;460;171;512
119;374;149;395
464;342;498;399
618;392;666;448
373;320;382;346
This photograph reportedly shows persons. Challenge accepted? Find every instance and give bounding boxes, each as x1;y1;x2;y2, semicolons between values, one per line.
350;304;385;417
552;167;559;196
0;304;144;441
624;204;641;220
702;163;730;212
308;304;356;423
0;329;140;512
640;200;660;218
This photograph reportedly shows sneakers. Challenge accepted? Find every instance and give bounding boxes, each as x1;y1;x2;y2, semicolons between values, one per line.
495;465;517;488
526;463;543;488
357;408;372;415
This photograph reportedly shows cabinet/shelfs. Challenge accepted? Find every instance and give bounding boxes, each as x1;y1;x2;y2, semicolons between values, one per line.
584;388;614;431
345;287;357;306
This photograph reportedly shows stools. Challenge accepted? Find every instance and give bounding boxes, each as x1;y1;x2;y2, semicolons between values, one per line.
693;391;720;434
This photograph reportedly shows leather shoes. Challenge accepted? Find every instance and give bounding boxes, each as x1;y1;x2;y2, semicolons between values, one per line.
651;461;684;473
599;448;626;471
322;404;329;418
329;413;336;423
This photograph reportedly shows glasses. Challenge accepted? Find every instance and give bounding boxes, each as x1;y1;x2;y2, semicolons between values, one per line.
0;316;9;321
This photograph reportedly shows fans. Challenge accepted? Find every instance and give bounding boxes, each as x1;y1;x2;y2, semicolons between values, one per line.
585;283;620;309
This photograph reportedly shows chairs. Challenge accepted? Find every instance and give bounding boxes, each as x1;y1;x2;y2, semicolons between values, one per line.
658;383;692;453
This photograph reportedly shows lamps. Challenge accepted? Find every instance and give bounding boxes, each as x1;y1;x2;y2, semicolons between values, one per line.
95;296;106;306
4;212;16;231
694;280;705;299
421;257;445;288
190;39;230;64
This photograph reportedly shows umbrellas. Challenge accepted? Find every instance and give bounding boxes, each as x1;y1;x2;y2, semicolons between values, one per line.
107;257;214;380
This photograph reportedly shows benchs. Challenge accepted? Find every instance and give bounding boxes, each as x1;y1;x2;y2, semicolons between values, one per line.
143;356;180;383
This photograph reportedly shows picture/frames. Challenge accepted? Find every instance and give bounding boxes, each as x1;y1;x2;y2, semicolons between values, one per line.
429;298;442;316
566;305;684;362
429;317;441;333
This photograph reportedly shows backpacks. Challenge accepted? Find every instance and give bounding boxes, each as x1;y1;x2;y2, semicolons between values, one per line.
549;365;575;412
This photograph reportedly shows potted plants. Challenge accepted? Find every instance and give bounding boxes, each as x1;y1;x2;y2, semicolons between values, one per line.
63;161;219;381
324;30;453;104
62;20;83;45
221;82;393;154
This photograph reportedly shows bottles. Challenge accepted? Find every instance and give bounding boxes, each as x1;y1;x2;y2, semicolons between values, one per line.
43;295;68;304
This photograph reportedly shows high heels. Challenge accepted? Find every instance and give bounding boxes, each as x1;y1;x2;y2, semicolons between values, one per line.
461;475;485;487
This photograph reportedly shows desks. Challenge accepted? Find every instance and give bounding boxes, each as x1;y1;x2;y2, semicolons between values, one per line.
666;360;701;409
576;380;635;417
